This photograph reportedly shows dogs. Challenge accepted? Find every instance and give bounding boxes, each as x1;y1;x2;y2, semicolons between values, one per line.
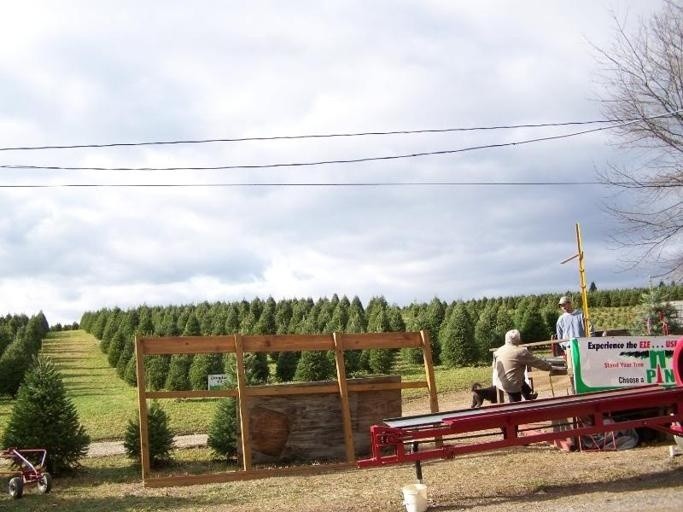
471;382;497;409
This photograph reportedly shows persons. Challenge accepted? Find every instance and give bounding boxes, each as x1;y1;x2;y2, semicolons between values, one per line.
554;295;596;393
492;329;553;402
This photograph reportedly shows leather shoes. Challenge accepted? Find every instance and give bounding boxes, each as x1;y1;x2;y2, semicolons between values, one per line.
525;393;538;400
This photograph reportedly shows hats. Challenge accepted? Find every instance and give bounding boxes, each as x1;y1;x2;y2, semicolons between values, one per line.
558;296;571;304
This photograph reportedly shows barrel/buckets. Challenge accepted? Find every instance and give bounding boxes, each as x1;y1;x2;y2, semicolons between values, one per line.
401;484;429;512
